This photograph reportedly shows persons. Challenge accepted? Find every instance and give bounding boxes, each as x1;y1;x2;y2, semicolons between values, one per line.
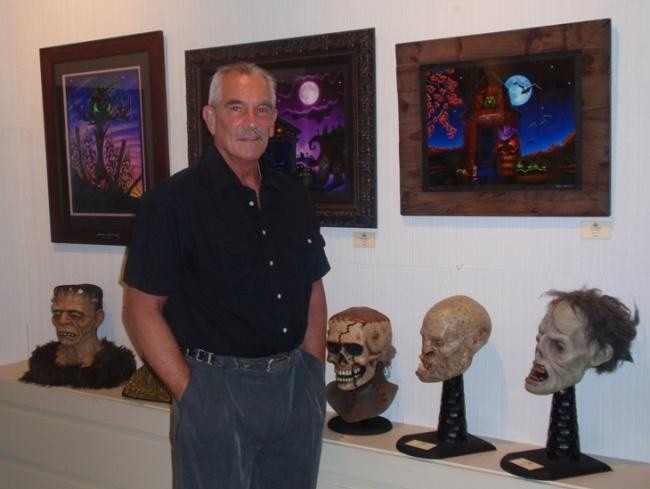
415;295;492;384
121;62;330;489
523;290;639;395
18;284;137;390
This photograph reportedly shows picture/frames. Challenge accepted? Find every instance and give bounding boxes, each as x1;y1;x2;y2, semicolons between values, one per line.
39;30;170;246
184;27;377;229
395;17;612;217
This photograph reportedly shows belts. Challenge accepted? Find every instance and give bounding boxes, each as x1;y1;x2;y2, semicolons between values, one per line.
180;345;296;374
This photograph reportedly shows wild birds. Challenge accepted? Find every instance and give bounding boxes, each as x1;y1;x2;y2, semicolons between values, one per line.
513;81;543;96
